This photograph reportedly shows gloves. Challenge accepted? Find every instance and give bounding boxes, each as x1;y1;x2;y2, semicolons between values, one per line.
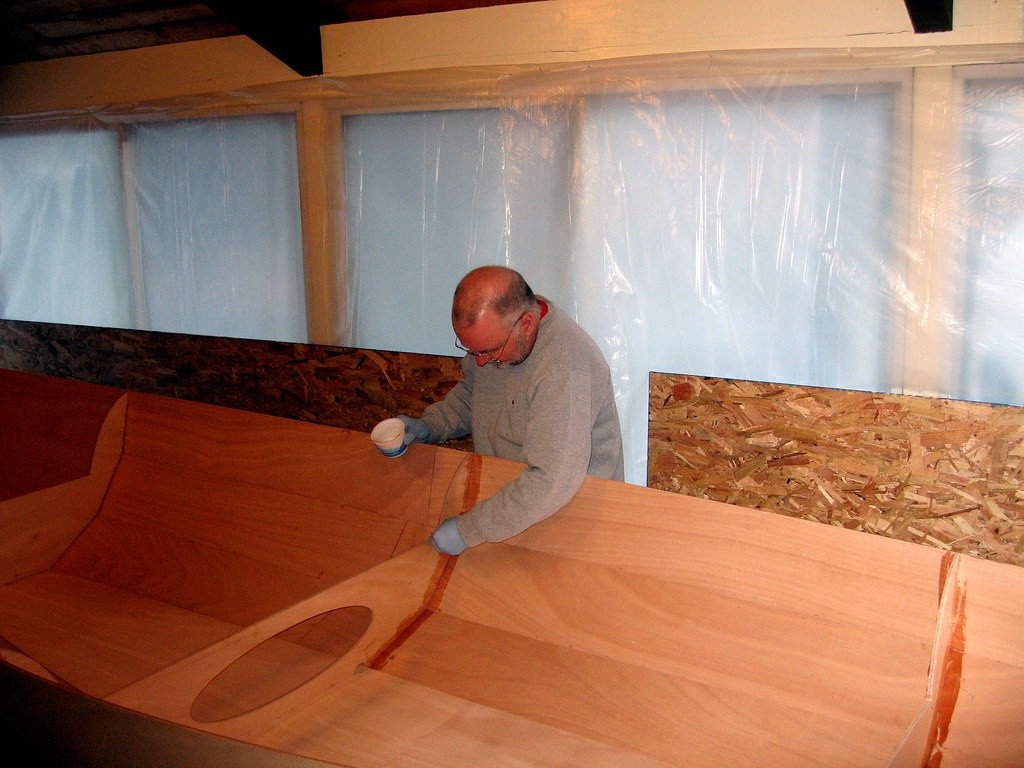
432;517;467;555
397;415;430;446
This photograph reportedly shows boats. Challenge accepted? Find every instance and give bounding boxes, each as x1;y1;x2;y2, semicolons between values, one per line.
1;365;1022;767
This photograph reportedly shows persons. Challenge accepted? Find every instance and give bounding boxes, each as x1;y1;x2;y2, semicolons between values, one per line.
396;265;623;556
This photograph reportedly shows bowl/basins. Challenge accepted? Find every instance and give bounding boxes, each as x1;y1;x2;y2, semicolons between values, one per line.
371;418;409;458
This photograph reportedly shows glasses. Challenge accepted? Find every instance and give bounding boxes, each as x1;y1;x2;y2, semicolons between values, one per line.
455;314;523;367
